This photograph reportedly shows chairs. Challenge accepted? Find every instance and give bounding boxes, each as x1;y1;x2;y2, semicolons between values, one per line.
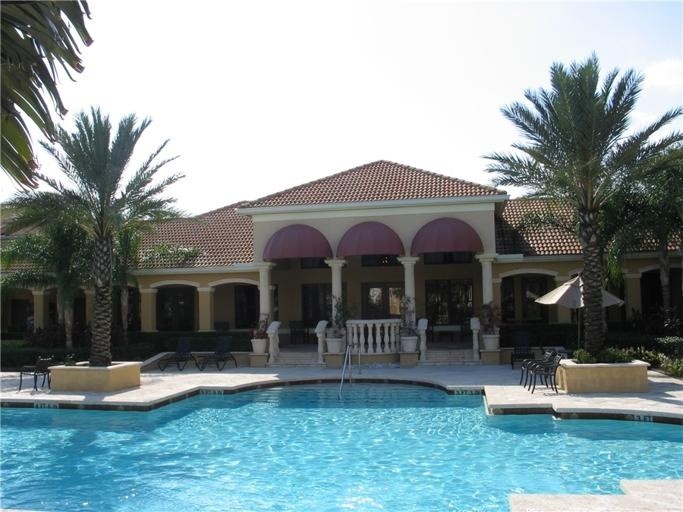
508;342;564;394
199;331;238;372
156;332;200;372
287;319;310;346
16;353;55;392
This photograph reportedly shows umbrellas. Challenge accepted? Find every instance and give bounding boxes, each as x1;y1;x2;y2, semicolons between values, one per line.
534;273;627;348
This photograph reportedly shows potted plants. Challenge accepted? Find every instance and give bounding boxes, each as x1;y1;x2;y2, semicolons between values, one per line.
245;310;275;353
390;293;420;353
322;289;361;354
472;298;515;350
